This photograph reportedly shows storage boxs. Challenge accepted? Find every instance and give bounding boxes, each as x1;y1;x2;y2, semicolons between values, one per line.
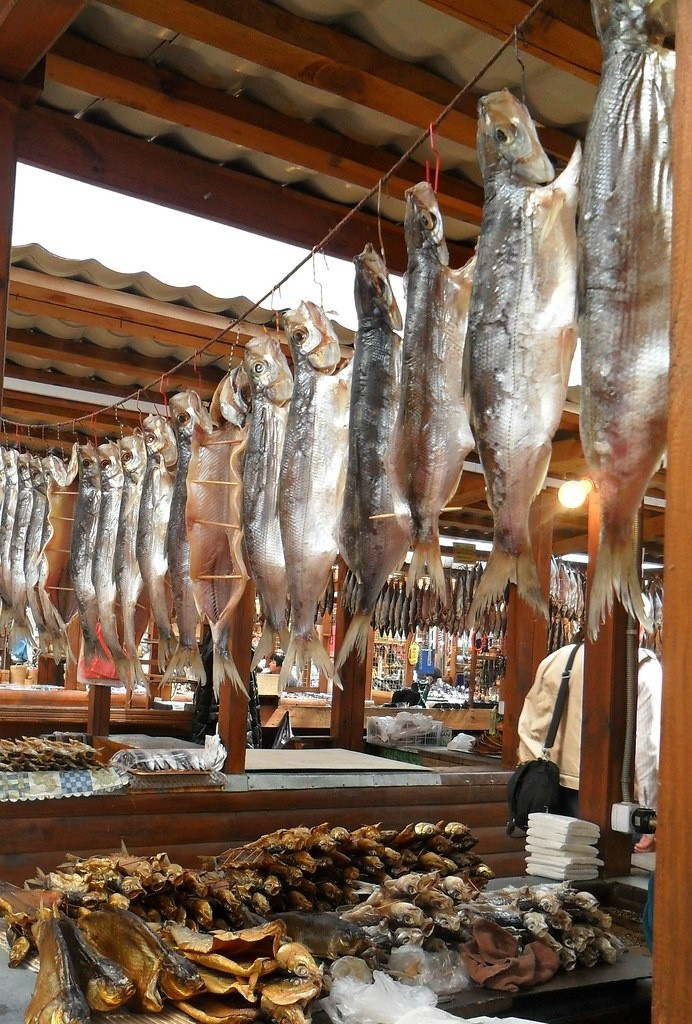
366;715;444;747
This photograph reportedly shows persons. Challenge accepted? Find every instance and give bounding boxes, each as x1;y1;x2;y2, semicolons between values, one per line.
421;668;444;708
518;617;663;856
189;627;263;750
264;653;299;686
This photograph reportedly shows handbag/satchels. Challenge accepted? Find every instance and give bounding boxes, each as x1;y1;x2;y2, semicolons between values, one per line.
510;758;562;830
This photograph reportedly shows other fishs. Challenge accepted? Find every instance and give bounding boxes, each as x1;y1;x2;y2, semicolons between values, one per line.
0;820;629;1024
0;0;678;704
2;733;219;773
